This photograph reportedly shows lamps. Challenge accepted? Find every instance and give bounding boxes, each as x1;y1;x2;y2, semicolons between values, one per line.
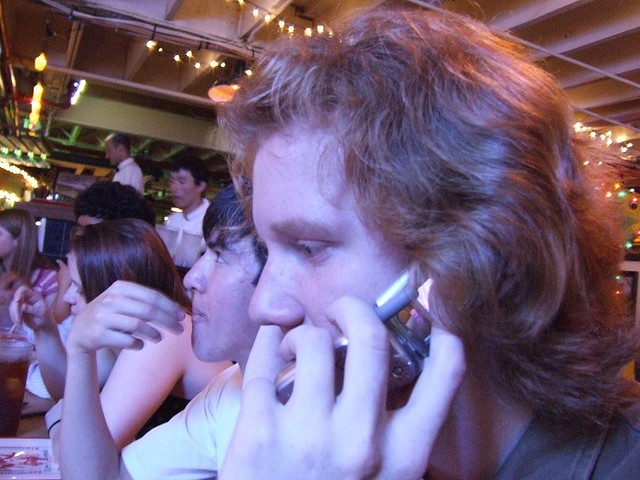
208;73;241;103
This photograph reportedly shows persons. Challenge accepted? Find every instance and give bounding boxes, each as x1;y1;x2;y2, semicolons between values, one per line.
59;181;284;480
23;182;159;404
104;133;144;197
1;207;58;341
154;154;214;277
8;216;238;468
218;0;638;480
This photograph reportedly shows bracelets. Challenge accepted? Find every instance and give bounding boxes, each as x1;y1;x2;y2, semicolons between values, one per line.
48;419;61;438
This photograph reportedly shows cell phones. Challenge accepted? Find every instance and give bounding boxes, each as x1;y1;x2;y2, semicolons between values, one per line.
274;264;431;406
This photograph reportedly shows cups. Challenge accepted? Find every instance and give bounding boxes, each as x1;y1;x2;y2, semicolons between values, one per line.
0;334;27;340
175;229;204;266
154;225;179;259
0;340;33;437
